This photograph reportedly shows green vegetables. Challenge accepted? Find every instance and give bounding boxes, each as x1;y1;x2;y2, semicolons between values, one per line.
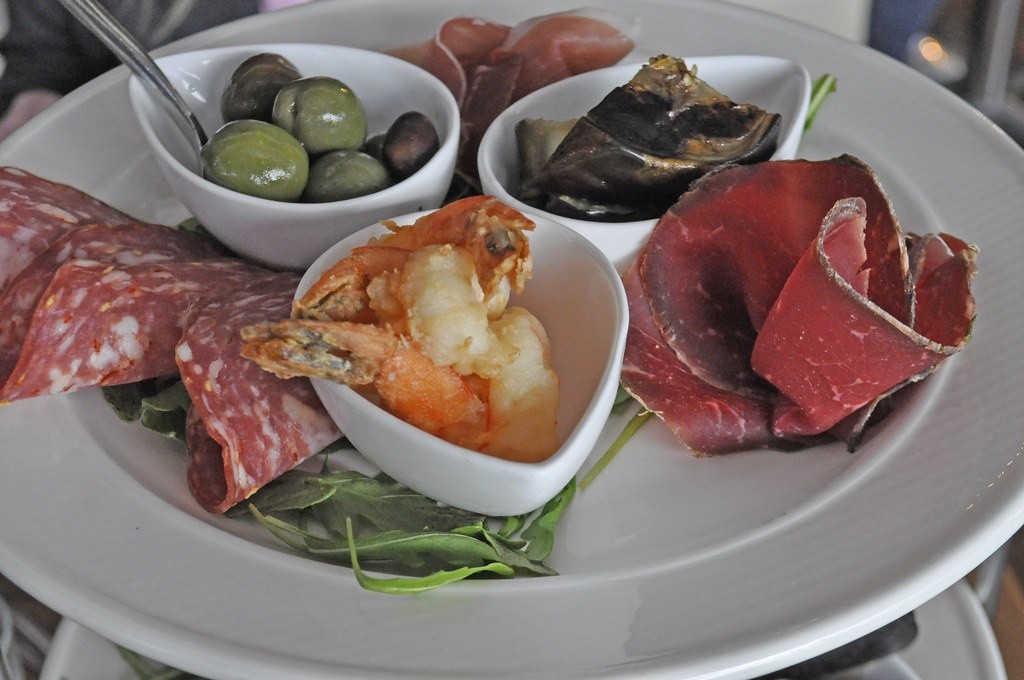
804;73;838;130
102;373;659;596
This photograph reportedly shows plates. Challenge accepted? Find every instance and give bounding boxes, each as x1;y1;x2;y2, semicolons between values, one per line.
0;0;1024;680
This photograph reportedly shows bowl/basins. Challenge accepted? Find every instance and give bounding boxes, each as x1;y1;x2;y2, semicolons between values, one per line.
477;55;813;278
290;208;630;518
128;42;462;270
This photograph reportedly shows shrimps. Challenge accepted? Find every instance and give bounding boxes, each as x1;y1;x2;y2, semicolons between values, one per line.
237;194;562;464
